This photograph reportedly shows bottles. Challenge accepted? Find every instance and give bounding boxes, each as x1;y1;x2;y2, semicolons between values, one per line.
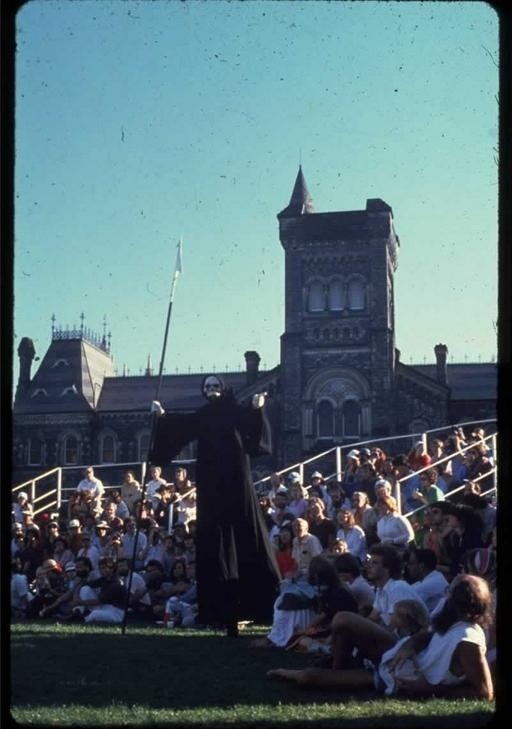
167;620;174;628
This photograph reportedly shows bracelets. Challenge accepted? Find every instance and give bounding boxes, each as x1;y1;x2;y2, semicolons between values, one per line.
431;685;435;698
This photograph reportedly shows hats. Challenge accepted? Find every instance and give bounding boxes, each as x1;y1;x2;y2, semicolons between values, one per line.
12;492;110;574
346;448;371;460
286;471;322;482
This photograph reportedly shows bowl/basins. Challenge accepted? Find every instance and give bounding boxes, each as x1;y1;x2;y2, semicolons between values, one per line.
170;618;181;625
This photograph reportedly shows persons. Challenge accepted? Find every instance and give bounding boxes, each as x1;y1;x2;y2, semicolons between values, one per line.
257;428;496;653
10;467;198;627
150;375;282;636
266;572;496;699
